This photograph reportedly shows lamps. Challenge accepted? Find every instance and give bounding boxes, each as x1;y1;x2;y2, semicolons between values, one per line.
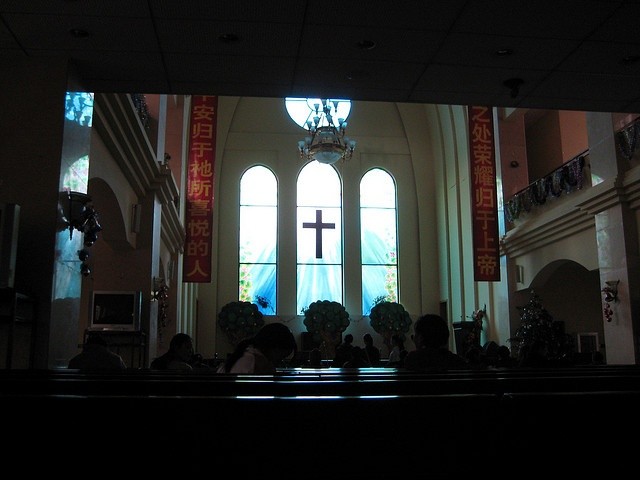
601;279;620;322
296;98;358;165
59;192;103;277
467;309;486;344
152;277;169;339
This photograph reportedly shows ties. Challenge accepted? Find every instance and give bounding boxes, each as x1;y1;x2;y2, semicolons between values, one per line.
88;288;143;330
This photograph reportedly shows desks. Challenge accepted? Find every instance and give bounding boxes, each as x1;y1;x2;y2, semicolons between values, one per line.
82;328;148;369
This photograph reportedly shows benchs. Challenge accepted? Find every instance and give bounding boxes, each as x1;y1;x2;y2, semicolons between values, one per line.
0;358;640;480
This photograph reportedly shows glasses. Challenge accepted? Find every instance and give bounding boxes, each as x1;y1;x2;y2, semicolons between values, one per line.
152;277;169;339
467;309;486;344
601;279;620;322
296;98;358;165
59;192;103;277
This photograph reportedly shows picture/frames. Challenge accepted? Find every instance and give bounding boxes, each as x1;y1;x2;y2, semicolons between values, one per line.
82;328;148;369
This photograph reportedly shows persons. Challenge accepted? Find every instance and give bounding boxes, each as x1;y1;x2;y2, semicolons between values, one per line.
335;334;354;360
344;346;367;366
363;333;380;364
150;333;193;368
67;335;128;368
404;314;455;368
388;335;403;366
593;351;604;361
216;323;297;375
496;346;514;366
